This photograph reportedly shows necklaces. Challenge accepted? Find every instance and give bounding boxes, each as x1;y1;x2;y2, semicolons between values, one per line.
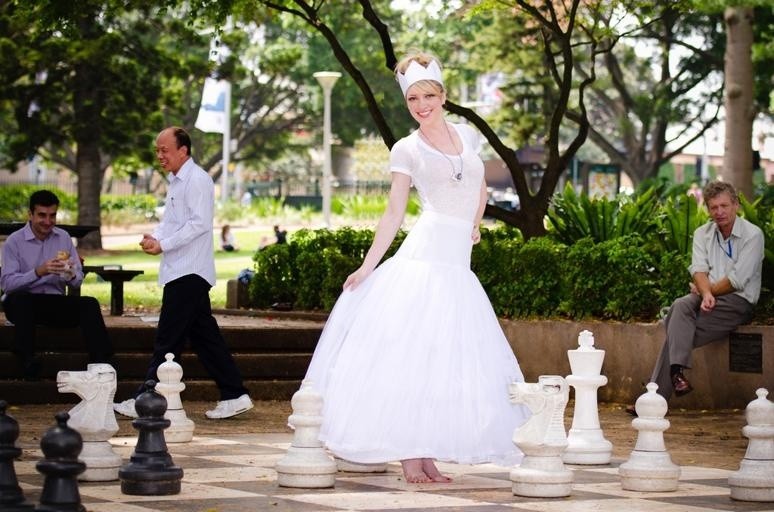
418;121;464;184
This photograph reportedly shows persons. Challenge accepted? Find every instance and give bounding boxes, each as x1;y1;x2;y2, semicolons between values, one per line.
687;183;703;208
113;126;254;418
624;180;768;416
220;225;238;252
287;51;535;482
0;190;122;383
273;225;281;246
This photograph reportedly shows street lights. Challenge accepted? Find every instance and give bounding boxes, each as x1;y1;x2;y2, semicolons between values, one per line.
311;68;344;227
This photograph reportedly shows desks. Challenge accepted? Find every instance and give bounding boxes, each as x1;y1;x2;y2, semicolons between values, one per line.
0;223;100;240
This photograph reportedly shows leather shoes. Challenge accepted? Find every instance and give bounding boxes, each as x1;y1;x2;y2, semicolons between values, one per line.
670;373;693;396
624;406;637;415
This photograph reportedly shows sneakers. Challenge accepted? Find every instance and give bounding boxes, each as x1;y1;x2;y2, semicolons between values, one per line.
205;393;253;419
113;398;139;418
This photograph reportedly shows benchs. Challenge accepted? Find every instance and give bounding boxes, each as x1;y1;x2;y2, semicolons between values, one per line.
64;266;144;316
